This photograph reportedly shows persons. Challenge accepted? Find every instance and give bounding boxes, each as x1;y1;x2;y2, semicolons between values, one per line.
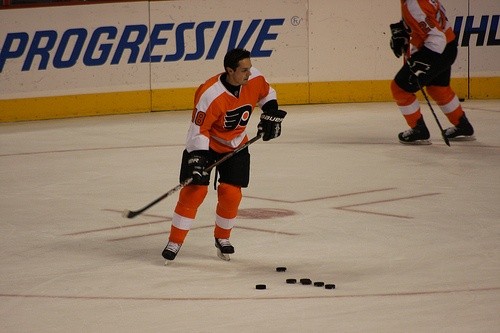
162;47;287;261
385;0;475;142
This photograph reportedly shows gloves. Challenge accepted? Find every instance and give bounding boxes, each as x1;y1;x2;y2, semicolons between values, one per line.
188;155;208;184
408;61;430;86
257;110;287;141
389;21;409;58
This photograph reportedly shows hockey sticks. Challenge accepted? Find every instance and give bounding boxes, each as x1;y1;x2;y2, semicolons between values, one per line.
400;47;451;147
120;131;265;219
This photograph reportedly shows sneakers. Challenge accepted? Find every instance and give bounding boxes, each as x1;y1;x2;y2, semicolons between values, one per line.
442;112;476;141
162;241;182;265
395;114;433;146
215;237;235;262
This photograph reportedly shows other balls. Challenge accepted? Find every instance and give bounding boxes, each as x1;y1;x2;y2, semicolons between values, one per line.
275;267;287;273
285;278;297;284
313;281;325;288
299;278;312;285
255;284;267;290
324;283;336;289
